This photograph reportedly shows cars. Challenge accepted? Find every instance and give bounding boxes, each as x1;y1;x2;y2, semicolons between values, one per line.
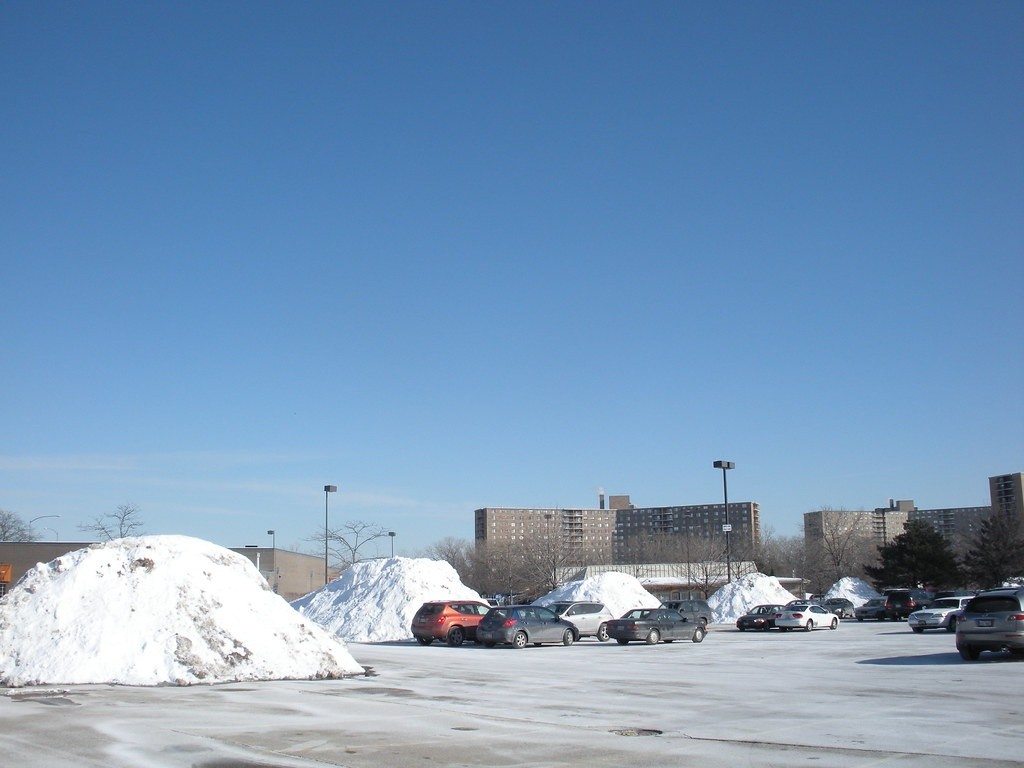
784;599;832;612
736;604;790;632
855;600;888;621
476;605;580;649
775;604;840;632
606;608;707;645
934;591;977;607
907;596;977;634
477;599;500;615
885;589;939;621
821;598;854;618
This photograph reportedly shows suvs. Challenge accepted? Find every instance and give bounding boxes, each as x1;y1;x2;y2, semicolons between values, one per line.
953;586;1024;661
410;601;502;647
532;599;615;641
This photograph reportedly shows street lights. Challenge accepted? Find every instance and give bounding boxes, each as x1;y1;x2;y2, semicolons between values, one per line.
388;531;396;558
27;514;62;542
267;530;275;549
324;485;337;585
43;527;59;542
713;460;735;583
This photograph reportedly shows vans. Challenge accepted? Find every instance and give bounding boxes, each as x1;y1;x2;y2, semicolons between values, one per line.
657;599;714;628
